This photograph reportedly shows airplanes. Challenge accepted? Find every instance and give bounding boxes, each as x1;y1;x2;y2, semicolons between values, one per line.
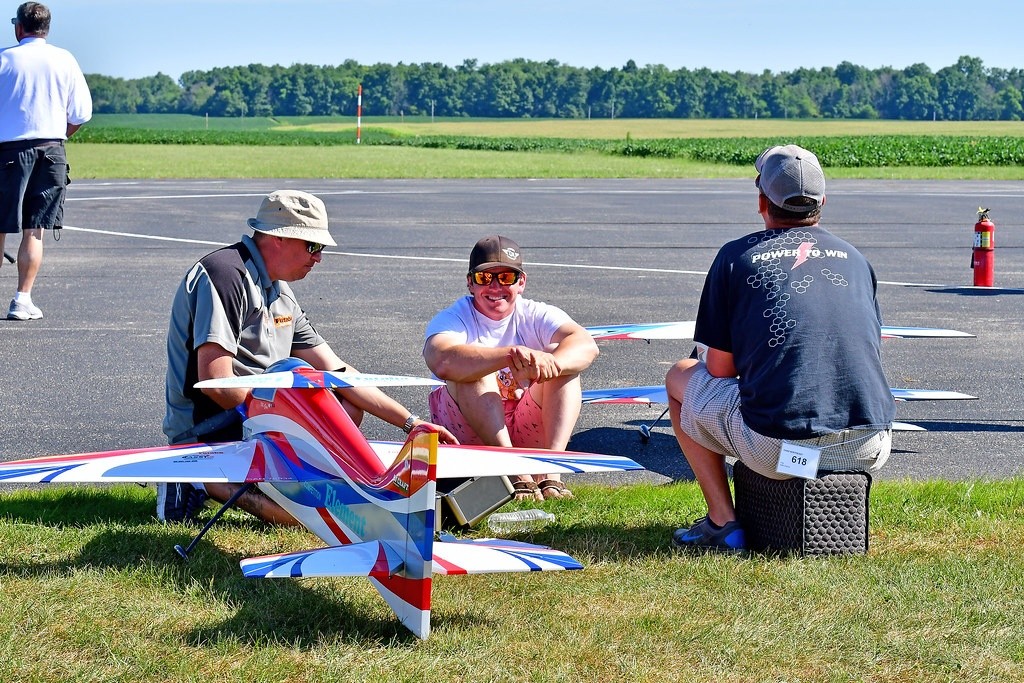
581;319;981;431
0;358;647;646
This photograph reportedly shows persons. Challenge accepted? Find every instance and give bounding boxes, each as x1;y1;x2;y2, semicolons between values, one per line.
666;145;898;551
154;189;599;522
0;1;93;319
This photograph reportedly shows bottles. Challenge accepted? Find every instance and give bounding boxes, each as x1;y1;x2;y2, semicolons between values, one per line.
487;509;555;534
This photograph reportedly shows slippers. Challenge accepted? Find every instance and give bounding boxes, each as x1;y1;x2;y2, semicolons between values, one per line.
537;480;575;499
513;481;544;501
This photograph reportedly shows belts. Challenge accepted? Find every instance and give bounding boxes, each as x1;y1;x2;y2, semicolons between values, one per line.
0;139;65;149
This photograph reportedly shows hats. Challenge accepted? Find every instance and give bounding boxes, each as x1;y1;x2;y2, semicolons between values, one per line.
247;190;338;246
468;235;523;275
12;2;51;29
755;145;825;212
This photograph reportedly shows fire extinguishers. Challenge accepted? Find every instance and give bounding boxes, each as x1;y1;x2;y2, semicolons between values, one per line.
971;206;995;287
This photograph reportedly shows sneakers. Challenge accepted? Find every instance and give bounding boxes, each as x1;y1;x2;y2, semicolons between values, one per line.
157;482;194;523
671;513;745;555
7;298;43;320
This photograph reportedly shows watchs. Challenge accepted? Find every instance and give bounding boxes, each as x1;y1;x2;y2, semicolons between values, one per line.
403;413;419;433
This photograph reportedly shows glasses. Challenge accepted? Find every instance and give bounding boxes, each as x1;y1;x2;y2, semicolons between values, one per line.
306;241;326;255
468;269;520;285
756;174;768;200
